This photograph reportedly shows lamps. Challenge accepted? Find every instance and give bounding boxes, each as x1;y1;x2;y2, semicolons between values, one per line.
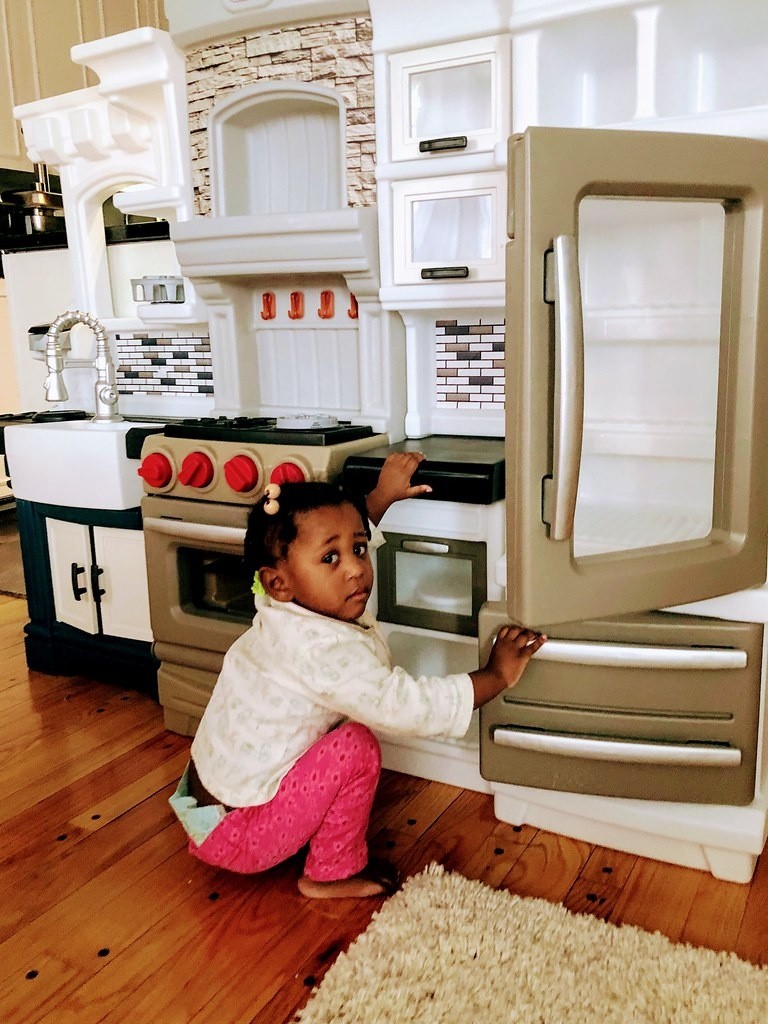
22;192;48;232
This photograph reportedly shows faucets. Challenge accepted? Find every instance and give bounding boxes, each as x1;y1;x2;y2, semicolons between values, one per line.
42;310;122;426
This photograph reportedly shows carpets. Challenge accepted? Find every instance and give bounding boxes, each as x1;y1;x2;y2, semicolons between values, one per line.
293;860;768;1024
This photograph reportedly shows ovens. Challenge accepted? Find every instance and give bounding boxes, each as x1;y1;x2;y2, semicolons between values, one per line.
141;492;256;734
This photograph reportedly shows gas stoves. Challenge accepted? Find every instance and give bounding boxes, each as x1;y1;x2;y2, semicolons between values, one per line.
145;413;387;511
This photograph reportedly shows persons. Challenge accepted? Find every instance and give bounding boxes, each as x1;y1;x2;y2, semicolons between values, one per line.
168;452;548;900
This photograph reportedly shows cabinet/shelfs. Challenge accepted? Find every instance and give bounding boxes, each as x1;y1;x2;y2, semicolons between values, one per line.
15;501;162;702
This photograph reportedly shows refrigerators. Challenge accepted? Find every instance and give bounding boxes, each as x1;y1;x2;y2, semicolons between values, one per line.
477;125;768;883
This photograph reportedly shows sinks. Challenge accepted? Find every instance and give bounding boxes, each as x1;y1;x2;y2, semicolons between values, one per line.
6;422;165;510
